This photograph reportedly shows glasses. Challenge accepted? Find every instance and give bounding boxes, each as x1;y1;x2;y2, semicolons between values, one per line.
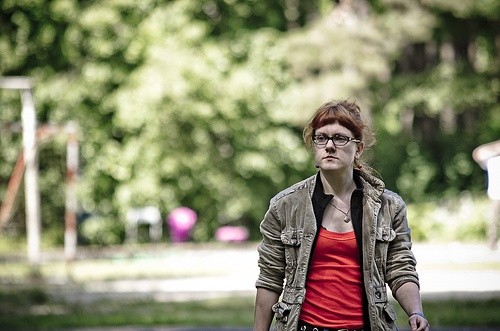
313;132;356;147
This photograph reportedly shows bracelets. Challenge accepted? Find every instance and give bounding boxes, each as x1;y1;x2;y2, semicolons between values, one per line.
408;312;427;319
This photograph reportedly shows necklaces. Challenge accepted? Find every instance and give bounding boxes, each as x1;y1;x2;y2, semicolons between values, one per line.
329;201;351;224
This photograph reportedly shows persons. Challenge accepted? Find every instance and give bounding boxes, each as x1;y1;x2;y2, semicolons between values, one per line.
252;97;434;331
